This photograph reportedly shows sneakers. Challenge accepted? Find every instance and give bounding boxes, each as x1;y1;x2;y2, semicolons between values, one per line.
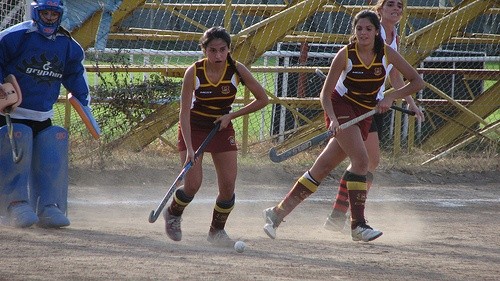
262;206;286;239
351;219;383;242
325;215;347;232
343;215;352;234
162;205;183;241
207;228;236;247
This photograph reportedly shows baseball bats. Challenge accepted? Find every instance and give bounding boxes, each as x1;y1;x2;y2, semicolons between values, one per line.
149;123;224;224
268;99;395;163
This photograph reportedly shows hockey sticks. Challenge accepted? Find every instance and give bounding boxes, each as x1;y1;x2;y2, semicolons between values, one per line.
314;67;416;118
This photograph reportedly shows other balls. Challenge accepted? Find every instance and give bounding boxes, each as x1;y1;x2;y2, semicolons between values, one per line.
234;240;246;253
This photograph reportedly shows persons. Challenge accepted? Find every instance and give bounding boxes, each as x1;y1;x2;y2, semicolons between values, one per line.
263;10;426;241
0;0;101;228
163;26;269;247
323;0;424;234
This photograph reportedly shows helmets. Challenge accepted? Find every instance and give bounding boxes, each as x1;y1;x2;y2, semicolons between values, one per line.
29;0;64;35
